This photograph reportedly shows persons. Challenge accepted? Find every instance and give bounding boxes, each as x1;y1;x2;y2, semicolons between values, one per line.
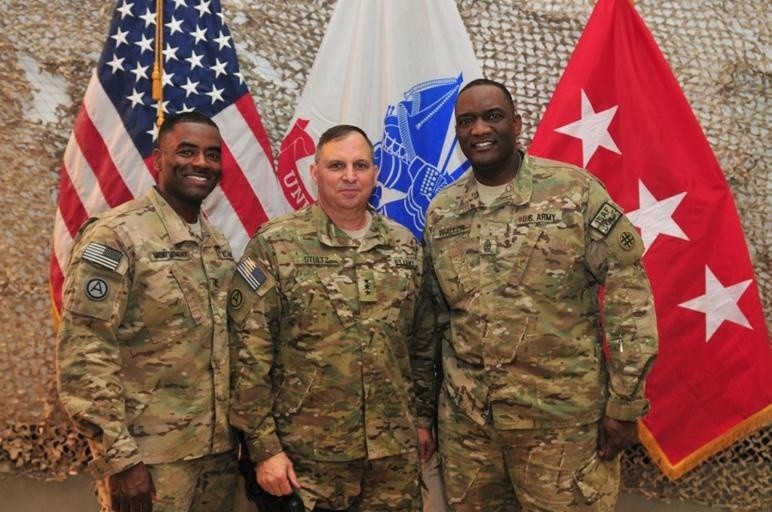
419;79;661;511
231;128;438;510
54;111;239;510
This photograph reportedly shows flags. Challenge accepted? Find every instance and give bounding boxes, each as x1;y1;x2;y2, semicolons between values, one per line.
528;2;770;481
275;0;489;241
46;0;275;336
